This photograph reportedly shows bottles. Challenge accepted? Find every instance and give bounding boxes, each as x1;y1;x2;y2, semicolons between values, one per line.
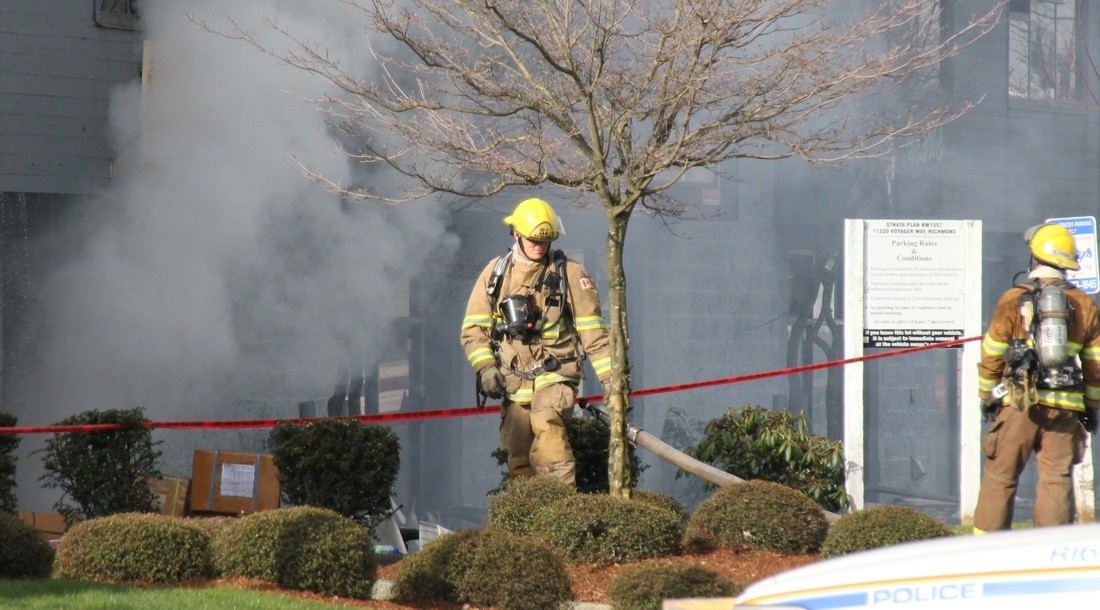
372;545;398;553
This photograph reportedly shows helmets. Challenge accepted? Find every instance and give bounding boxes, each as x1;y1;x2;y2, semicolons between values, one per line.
1029;223;1080;270
503;199;561;242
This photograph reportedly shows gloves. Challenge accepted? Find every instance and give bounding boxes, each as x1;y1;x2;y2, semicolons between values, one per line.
600;382;611;404
478;365;506;399
981;398;1001;422
1092;409;1100;435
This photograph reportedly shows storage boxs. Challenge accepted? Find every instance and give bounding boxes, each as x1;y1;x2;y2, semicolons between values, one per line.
189;450;281;515
133;473;190;516
15;512;81;543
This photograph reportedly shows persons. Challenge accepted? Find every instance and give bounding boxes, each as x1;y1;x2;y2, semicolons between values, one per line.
973;225;1100;537
460;198;612;494
